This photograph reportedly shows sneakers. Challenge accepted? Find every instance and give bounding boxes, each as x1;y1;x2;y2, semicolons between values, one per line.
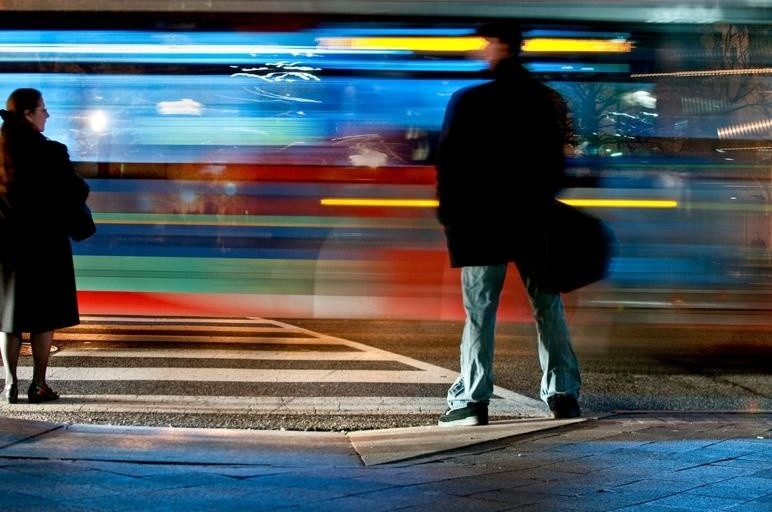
547;394;582;419
437;403;488;429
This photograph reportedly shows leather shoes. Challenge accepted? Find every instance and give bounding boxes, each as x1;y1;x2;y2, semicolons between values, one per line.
3;384;18;404
27;384;58;403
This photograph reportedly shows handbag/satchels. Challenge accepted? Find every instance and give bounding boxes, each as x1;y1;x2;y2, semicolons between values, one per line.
524;201;615;291
68;205;96;242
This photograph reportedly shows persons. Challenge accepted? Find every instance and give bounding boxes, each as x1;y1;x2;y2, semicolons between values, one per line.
433;20;582;427
749;230;767;250
0;88;95;403
